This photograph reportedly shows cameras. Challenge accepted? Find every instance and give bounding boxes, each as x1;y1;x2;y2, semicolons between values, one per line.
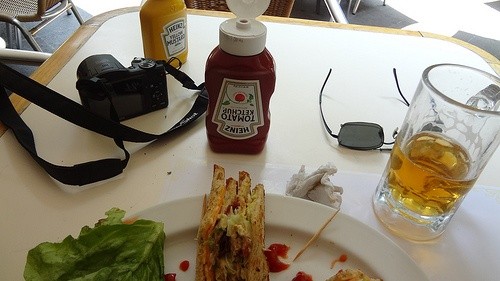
75;54;168;123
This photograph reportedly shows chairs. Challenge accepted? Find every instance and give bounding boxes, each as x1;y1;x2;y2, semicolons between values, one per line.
0;47;53;97
183;0;349;23
0;0;85;52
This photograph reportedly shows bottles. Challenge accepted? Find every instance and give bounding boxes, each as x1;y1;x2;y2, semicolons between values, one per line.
205;0;277;155
138;0;188;68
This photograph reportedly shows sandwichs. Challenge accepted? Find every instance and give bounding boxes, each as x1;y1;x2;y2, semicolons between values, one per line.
191;164;269;281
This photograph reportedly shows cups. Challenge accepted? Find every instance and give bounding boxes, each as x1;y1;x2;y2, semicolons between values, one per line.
372;64;500;244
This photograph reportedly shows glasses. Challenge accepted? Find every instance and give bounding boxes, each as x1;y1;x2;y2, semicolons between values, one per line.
320;67;442;150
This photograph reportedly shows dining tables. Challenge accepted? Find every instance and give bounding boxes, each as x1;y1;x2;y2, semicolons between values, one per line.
0;4;500;281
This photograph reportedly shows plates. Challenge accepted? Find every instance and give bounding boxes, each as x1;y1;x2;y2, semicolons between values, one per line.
119;191;427;281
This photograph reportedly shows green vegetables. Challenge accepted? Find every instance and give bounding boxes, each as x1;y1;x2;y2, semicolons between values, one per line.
23;207;166;281
